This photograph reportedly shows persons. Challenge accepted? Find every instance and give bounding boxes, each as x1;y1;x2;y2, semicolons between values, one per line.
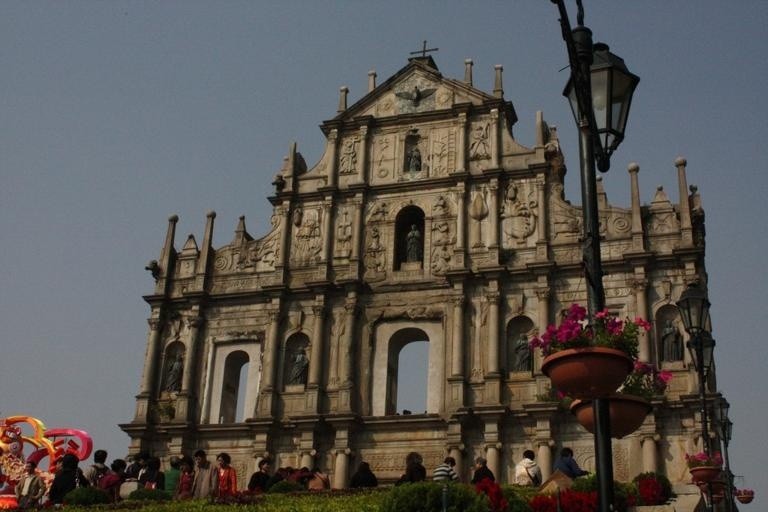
164;352;185;394
363;251;386;279
552;446;593;484
339;139;357;174
515;450;542;486
369;226;380;249
286;346;311;386
659;319;685;363
14;460;47;512
292;210;323;267
406;146;422;172
432;220;450;245
368;202;388;223
432;195;451;216
466;121;491;159
247;459;331;494
394;452;427;486
470;456;496;486
432;456;460;483
513;327;531;372
430;246;451;274
404;223;423;263
49;450;238;505
348;461;378;487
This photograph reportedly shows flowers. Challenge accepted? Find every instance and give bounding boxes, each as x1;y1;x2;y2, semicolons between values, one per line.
733;488;754;496
684;451;726;469
525;298;651;360
640;478;675;506
539;364;674;404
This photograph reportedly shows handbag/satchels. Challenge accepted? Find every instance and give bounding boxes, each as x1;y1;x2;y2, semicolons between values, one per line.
74;468;91;490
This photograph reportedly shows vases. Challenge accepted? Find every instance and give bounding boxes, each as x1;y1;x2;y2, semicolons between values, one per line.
713;494;723;502
737;496;754;503
635;505;676;512
574;400;648;440
538;348;636;400
689;466;719;481
699;481;722;494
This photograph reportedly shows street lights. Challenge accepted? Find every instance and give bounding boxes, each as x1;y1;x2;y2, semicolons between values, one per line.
676;280;718;509
551;1;638;510
710;391;738;511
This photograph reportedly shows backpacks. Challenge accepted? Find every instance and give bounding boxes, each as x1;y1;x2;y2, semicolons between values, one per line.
90;464;109;488
